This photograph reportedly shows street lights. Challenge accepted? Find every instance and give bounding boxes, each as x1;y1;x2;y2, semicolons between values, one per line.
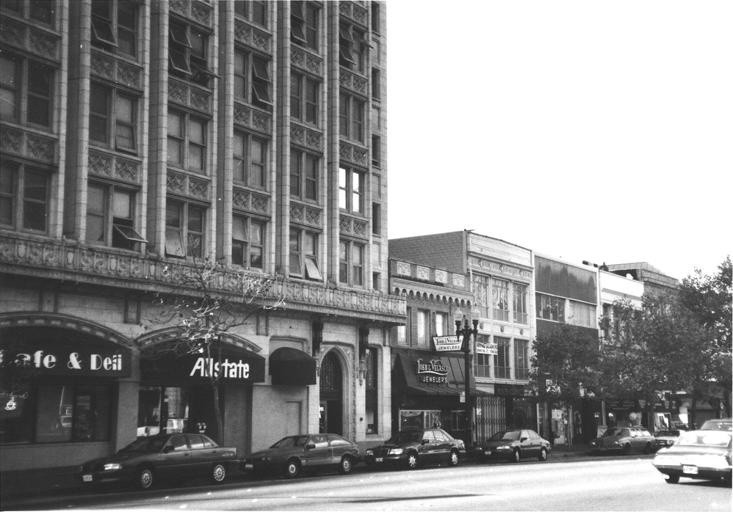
454;306;481;428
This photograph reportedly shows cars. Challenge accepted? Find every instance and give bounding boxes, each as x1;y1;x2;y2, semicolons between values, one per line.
245;434;361;478
72;433;244;491
652;419;732;485
363;428;468;469
591;426;658;455
655;429;686;450
475;429;551;462
